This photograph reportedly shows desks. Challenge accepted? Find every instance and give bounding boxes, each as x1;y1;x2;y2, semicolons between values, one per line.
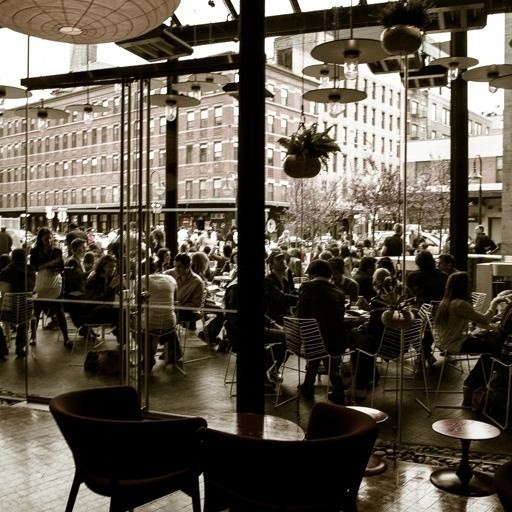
198;412;305;441
427;418;502;497
343;406;390;474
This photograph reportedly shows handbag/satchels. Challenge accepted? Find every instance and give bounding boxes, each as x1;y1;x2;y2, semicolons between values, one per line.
33;269;63;299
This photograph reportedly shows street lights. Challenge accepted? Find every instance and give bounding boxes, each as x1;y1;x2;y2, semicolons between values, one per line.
467;154;483;230
151;170;166;225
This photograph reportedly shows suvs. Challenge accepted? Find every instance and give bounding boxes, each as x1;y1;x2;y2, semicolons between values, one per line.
8;228;120;248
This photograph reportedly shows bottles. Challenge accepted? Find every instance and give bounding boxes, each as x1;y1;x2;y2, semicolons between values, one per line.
344;295;366;313
120;274;138;293
204;280;228;295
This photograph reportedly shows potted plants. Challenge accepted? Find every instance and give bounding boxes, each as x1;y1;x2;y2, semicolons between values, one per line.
271;117;345;182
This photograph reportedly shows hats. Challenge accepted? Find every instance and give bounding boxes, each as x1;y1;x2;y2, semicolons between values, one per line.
304;259;332;277
326;257;345;273
265;247;285;265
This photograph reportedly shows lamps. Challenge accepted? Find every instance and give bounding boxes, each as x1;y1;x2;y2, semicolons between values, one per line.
0;0;512;137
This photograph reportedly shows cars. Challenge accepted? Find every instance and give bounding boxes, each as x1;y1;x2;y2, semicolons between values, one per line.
278;224;449;256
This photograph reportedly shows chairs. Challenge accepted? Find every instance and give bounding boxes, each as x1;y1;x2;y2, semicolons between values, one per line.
49;386;207;511
0;235;512;431
176;403;377;511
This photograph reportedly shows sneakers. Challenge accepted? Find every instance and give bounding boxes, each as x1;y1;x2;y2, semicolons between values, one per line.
269;368;283;383
15;345;26;358
29;335;37;346
262;376;277;389
297;384;315;402
198;331;221;345
327;392;345;404
142;355;156;374
64;337;74;348
